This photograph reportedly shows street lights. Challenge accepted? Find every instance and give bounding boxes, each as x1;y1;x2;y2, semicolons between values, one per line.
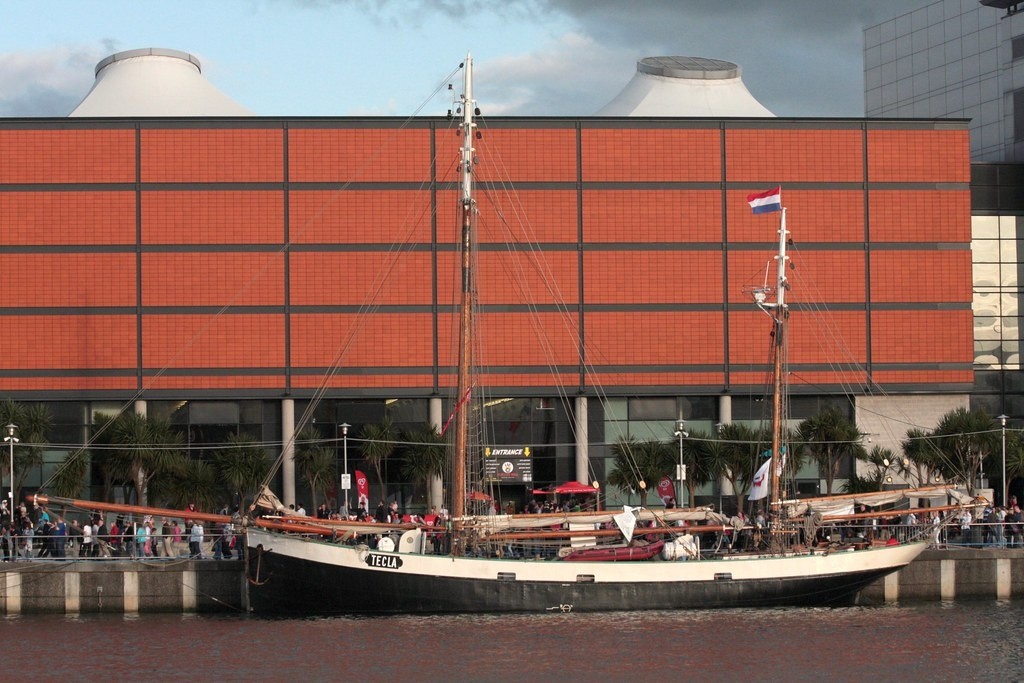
671;419;689;507
6;423;19;537
997;414;1009;510
338;422;352;522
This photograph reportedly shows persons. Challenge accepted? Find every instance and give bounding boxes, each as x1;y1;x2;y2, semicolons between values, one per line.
0;500;282;561
735;496;1024;553
289;495;597;556
664;497;676;542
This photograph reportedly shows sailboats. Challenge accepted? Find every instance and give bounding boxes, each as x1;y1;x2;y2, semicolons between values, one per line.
236;50;963;618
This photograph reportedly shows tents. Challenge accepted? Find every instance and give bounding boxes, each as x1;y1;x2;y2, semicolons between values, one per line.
531;481;600;512
464;492;494;516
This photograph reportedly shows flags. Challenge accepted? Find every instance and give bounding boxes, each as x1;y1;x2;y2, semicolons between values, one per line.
746;186;781;214
748;453;786;501
441;385;474;436
759;446;787;457
